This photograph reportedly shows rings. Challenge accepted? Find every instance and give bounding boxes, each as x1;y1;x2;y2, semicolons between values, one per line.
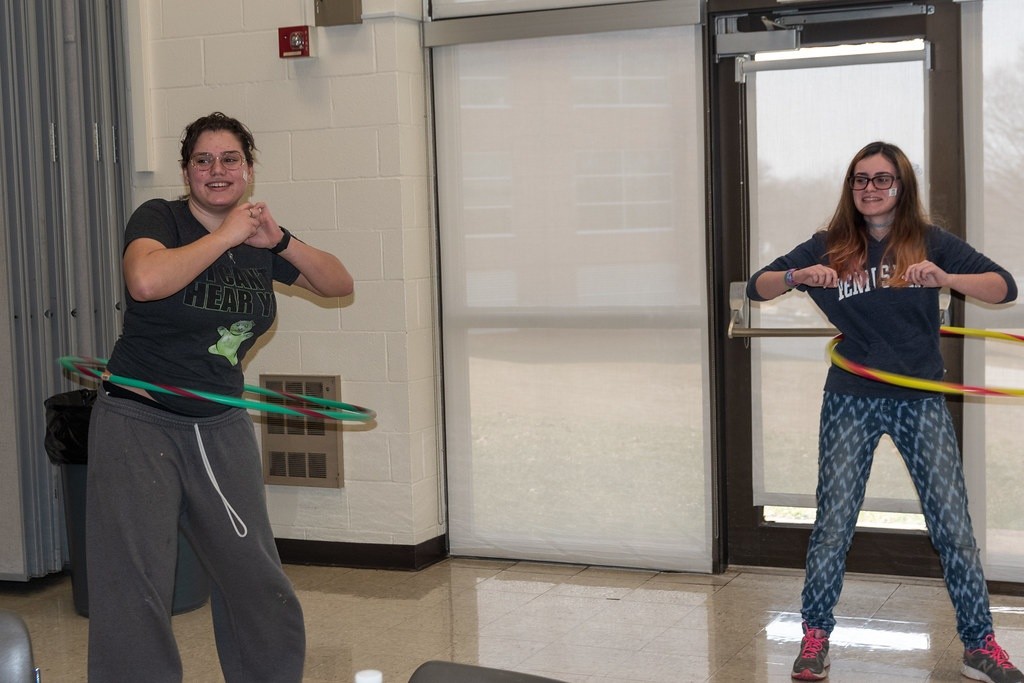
249;208;255;217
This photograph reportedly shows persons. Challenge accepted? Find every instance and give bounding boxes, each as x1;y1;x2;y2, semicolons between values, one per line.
83;110;354;683
746;141;1024;683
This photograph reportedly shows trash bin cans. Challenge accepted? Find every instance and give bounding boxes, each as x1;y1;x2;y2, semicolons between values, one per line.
43;387;211;620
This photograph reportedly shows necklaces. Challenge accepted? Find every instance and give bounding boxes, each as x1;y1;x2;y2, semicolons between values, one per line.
227;249;236;265
866;221;895;227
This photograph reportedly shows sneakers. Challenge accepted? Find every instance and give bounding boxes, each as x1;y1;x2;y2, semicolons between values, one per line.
961;634;1024;683
791;621;830;680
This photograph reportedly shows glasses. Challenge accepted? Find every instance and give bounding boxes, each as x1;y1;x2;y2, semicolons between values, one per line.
849;175;901;190
188;150;247;171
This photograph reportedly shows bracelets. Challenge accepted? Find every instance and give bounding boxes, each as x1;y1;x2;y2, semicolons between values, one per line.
784;268;800;291
271;226;292;254
